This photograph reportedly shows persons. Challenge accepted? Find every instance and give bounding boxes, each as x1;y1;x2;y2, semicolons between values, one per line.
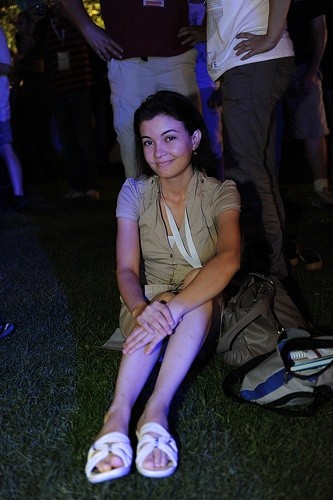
84;89;243;483
287;0;333;205
0;0;117;211
188;0;224;0
189;1;224;179
62;1;206;182
205;0;295;280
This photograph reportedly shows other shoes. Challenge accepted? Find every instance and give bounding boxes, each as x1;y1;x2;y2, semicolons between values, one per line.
313;181;332;206
8;196;28;210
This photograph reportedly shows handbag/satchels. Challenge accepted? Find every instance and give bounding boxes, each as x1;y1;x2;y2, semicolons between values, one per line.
216;270;333;416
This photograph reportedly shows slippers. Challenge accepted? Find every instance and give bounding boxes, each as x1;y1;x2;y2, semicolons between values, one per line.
135;423;178;478
84;415;133;484
